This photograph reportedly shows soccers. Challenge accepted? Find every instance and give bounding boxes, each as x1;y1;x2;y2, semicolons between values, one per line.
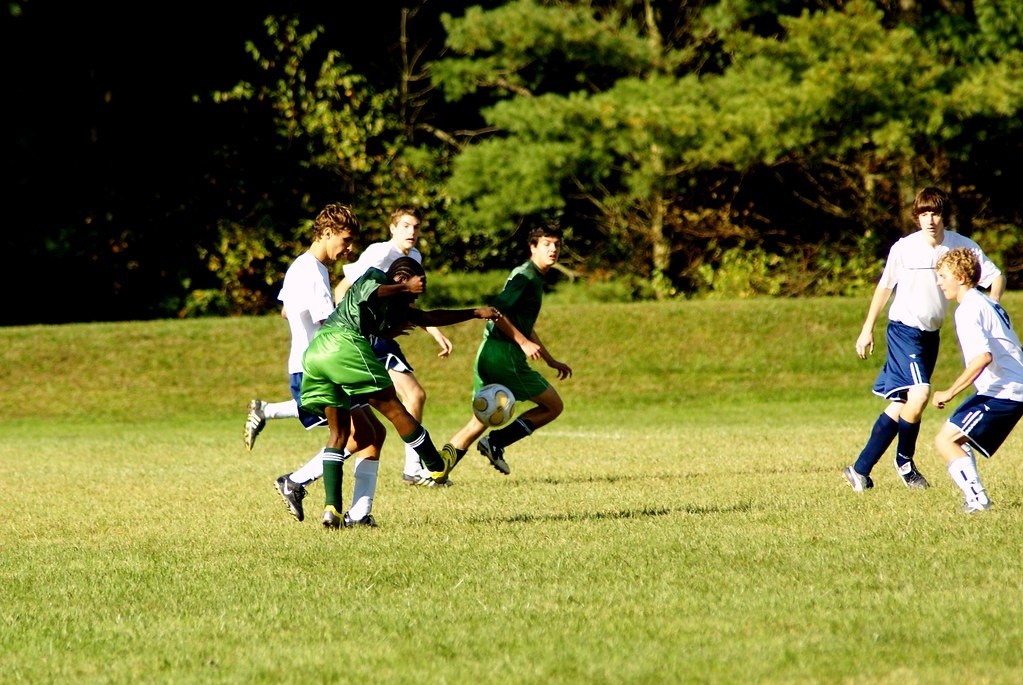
472;382;516;428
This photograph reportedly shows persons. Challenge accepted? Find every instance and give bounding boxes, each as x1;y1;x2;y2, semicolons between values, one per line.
933;247;1023;515
333;206;454;528
242;203;376;522
841;187;1008;498
298;257;504;530
448;223;572;475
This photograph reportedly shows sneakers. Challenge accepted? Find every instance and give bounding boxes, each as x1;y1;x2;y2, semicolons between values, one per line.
273;472;308;523
241;399;266;452
321;505;344;530
958;497;991;514
342;511;377;528
893;456;930;490
476;435;511;475
841;465;875;493
429;442;458;485
402;472;454;488
